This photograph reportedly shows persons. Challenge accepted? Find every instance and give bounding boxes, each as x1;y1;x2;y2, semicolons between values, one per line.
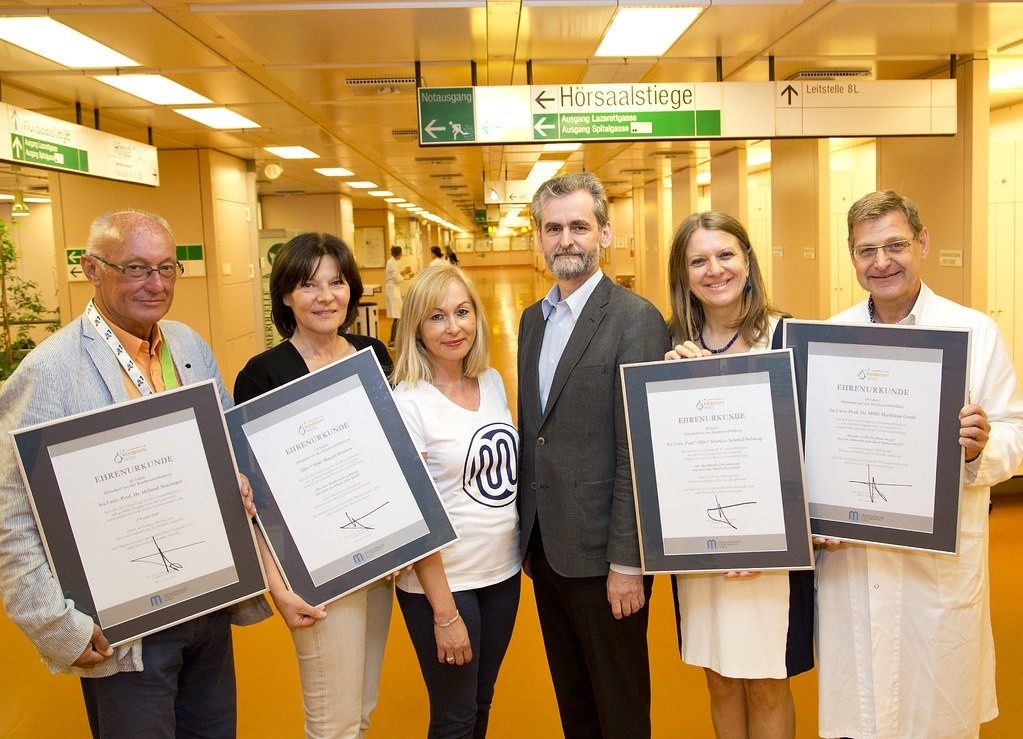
385;246;415;350
517;171;672;739
234;232;412;739
814;191;1023;739
663;212;815;739
388;264;522;739
0;210;274;739
428;245;459;266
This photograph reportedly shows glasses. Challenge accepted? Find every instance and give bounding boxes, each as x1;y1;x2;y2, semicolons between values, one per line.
91;252;186;282
851;234;915;262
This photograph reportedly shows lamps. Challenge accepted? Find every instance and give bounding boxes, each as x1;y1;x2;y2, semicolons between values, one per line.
264;157;283;179
11;164;30;216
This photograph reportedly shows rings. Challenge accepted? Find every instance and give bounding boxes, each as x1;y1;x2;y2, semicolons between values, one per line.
445;657;454;662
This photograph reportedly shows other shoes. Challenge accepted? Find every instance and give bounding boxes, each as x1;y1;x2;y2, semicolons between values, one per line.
388;342;395;350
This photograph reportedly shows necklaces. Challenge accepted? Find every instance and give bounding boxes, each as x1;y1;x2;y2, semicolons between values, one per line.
699;326;740;353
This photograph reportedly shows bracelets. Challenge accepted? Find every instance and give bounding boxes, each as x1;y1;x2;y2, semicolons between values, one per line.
433;610;459;627
